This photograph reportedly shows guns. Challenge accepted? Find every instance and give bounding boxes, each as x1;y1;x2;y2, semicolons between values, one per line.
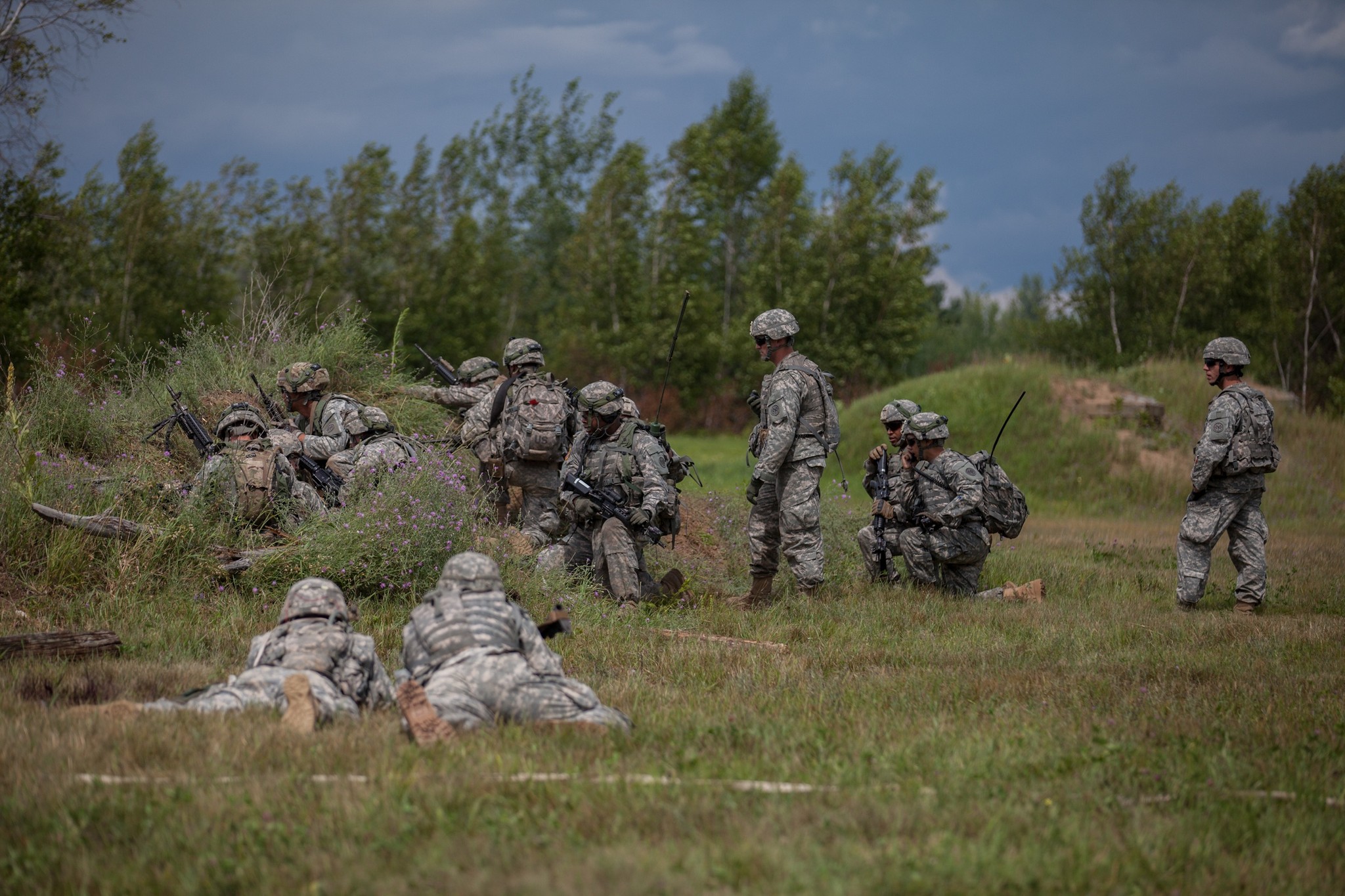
139;384;217;459
251;372;287;432
537;601;575;639
299;454;348;505
414;344;460;387
910;502;936;534
874;449;888;562
561;473;666;549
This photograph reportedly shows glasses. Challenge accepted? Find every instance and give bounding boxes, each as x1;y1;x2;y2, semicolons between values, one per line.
906;437;916;446
1205;358;1217;367
884;422;903;432
754;336;767;346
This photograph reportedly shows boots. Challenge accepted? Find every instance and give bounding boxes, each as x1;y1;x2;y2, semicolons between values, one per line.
1003;578;1046;604
619;568;816;610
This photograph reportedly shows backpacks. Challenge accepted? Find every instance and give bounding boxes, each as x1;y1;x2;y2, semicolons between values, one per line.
643;420;703;550
966;450;1030;543
215;446;283;522
498;373;572;461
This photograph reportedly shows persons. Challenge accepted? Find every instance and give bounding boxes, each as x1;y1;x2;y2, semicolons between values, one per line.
856;399;922;587
395;550;633;748
392;335;702;615
900;411;1047;605
1174;337;1279;618
723;308;841;610
79;577;389;752
189;363;421;543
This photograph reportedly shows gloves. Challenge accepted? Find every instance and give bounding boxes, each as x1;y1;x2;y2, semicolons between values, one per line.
747;389;761;414
625;507;650;526
574;496;601;521
746;477;763;505
871;500;893;520
868;443;887;460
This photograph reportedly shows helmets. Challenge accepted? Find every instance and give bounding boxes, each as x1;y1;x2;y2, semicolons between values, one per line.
902;412;949;440
880;397;921;423
435;552;504;594
457;337;642;420
280;579;354;623
213;361;402;456
1203;337;1251;366
750;309;799;340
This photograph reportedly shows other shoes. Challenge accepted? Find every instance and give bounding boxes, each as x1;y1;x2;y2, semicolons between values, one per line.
1233;599;1255;616
277;675;319;738
503;523;535;559
396;680;459;749
